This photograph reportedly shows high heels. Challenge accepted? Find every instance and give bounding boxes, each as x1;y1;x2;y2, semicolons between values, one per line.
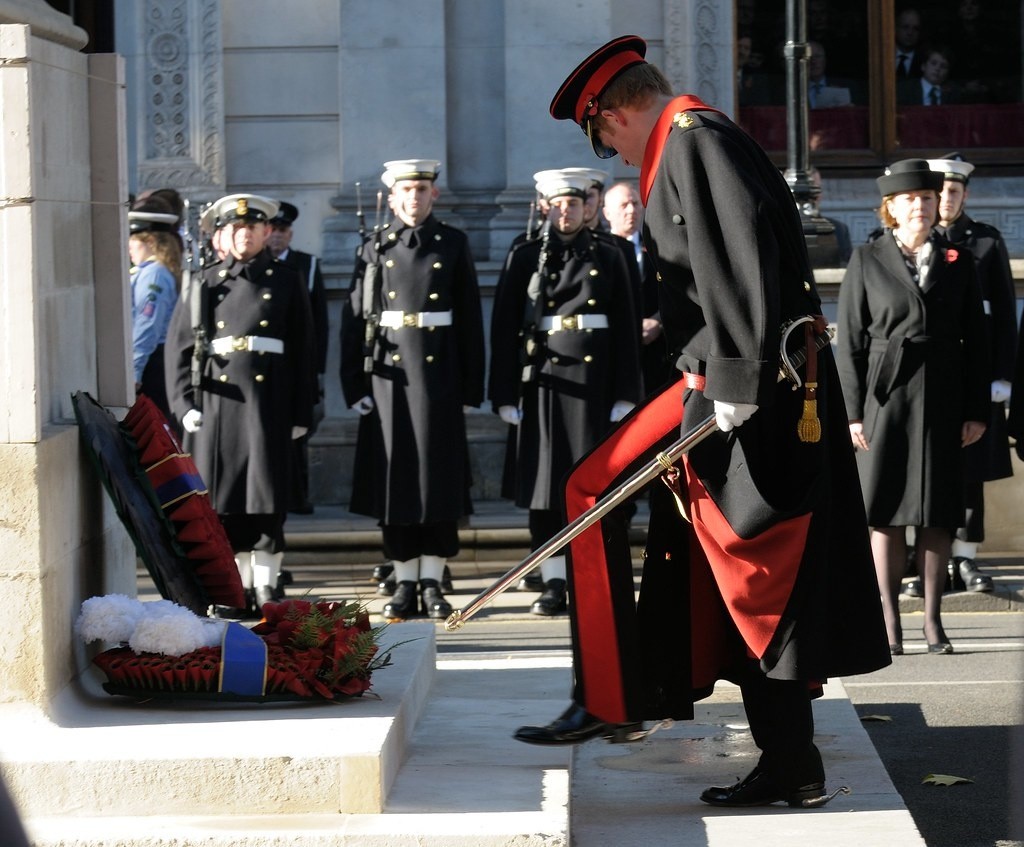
922;624;953;654
888;627;904;655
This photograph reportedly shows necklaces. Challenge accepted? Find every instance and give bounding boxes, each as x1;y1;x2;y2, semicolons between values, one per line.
897;232;927;255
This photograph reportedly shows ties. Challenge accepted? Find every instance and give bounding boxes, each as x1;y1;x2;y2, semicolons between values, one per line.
896;54;910;77
929;87;938;105
813;83;822;99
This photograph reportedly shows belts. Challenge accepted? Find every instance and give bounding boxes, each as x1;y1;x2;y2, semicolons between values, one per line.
207;335;284;355
539;313;610;331
379;310;453;328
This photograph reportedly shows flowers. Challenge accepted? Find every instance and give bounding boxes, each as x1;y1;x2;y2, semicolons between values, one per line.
74;586;410;700
944;249;958;262
120;395;248;612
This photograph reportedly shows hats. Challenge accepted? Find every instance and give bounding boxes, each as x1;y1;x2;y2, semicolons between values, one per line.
876;152;979;196
129;187;184;234
380;159;441;188
199;193;299;235
533;167;611;204
549;33;648;161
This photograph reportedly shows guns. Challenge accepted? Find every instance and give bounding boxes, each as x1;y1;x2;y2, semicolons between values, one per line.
510;196;558;421
356;185;394;413
174;196;215;451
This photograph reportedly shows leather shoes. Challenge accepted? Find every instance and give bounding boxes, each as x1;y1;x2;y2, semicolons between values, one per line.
952;559;995;593
370;561;453;618
512;701;677;745
700;760;852;808
530;578;567;615
900;559;924;597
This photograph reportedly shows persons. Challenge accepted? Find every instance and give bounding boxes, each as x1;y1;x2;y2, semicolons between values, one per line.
164;193;321;609
837;152;1014;655
487;167;683;616
339;159;485;619
511;35;891;809
892;8;978;151
1006;310;1024;462
128;187;191;441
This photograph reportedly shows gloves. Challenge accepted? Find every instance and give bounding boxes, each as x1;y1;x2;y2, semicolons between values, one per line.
714;399;759;432
499;405;523;425
610;401;636;423
291;426;307;440
991;380;1012;402
351;396;372;415
181;408;204;433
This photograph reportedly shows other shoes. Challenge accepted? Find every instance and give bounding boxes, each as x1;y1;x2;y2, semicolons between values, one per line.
517;575;545;591
213;566;294;619
